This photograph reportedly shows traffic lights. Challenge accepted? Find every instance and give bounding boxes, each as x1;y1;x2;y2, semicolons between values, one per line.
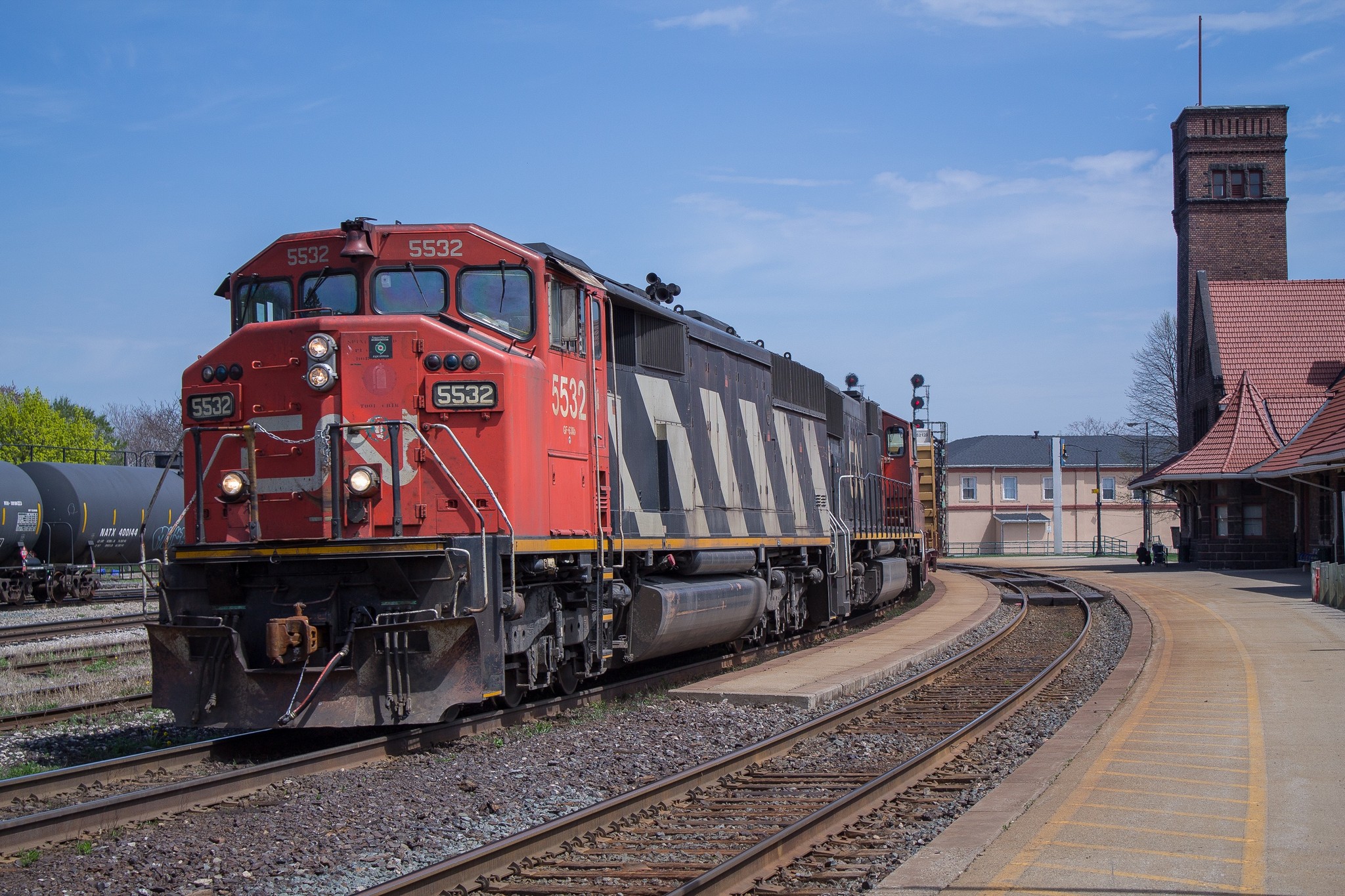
912;419;925;429
910;374;925;388
910;396;925;410
845;372;858;387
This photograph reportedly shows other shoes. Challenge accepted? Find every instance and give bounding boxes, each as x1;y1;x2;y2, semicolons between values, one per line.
1145;563;1147;566
1139;562;1142;566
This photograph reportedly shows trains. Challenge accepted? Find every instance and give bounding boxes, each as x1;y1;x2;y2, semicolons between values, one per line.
0;444;186;606
136;217;932;735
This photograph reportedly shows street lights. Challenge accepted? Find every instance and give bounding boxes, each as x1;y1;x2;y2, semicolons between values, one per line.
1058;444;1104;556
1126;421;1152;547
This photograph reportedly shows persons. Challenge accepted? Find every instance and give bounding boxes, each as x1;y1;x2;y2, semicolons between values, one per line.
1135;542;1150;566
